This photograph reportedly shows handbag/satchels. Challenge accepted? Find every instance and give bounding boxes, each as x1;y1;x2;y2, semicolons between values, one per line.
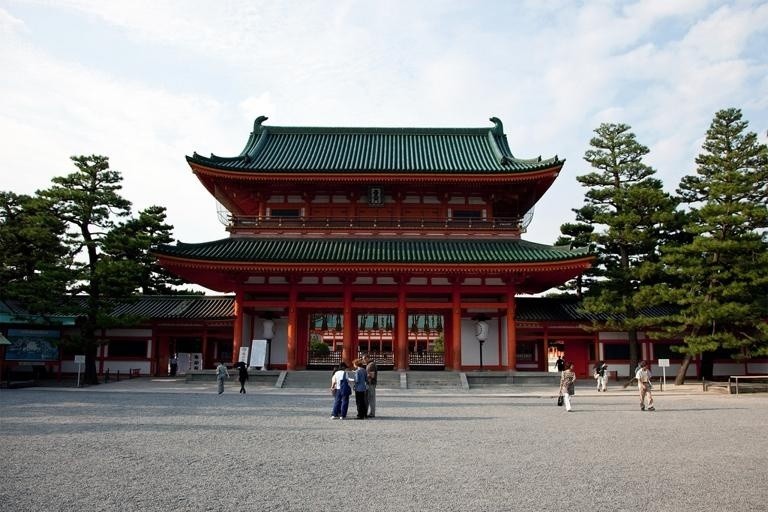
339;378;353;395
558;395;564;406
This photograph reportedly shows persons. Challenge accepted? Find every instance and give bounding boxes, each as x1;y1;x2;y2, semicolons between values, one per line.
597;361;605;391
558;361;577;412
351;358;367;420
330;362;355;421
554;355;565;370
215;359;230;395
635;363;641;376
635;360;655;412
601;365;609;393
168;351;179;378
330;366;342;414
362;354;377;417
238;362;249;394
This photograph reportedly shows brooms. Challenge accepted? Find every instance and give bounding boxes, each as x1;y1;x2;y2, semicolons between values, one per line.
623;377;635;388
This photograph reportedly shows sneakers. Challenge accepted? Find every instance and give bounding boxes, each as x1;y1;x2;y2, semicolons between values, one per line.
332;415;346;420
641;407;655;411
